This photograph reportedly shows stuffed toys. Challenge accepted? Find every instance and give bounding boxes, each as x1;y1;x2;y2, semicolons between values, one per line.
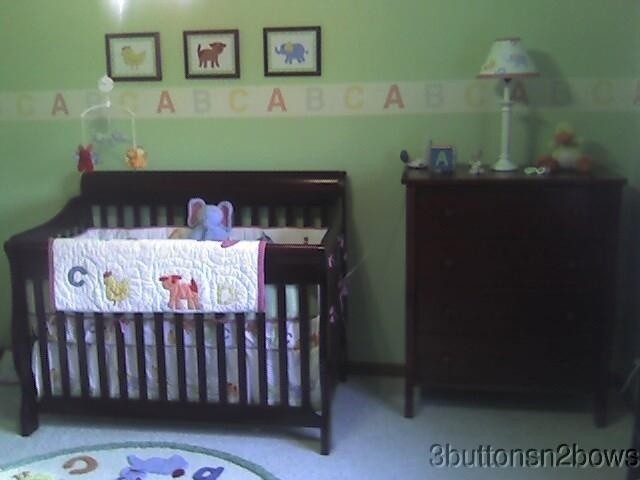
540;129;596;170
185;196;234;241
75;142;97;173
92;128;127;148
123;146;146;171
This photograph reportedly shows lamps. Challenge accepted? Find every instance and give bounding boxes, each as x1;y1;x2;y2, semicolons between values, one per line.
475;36;542;170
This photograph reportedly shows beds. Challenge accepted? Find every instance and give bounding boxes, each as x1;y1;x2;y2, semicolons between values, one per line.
3;169;350;456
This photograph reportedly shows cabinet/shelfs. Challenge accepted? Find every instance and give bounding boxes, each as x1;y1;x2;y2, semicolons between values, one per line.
402;162;629;429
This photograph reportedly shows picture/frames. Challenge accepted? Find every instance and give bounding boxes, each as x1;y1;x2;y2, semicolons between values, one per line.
105;27;322;82
